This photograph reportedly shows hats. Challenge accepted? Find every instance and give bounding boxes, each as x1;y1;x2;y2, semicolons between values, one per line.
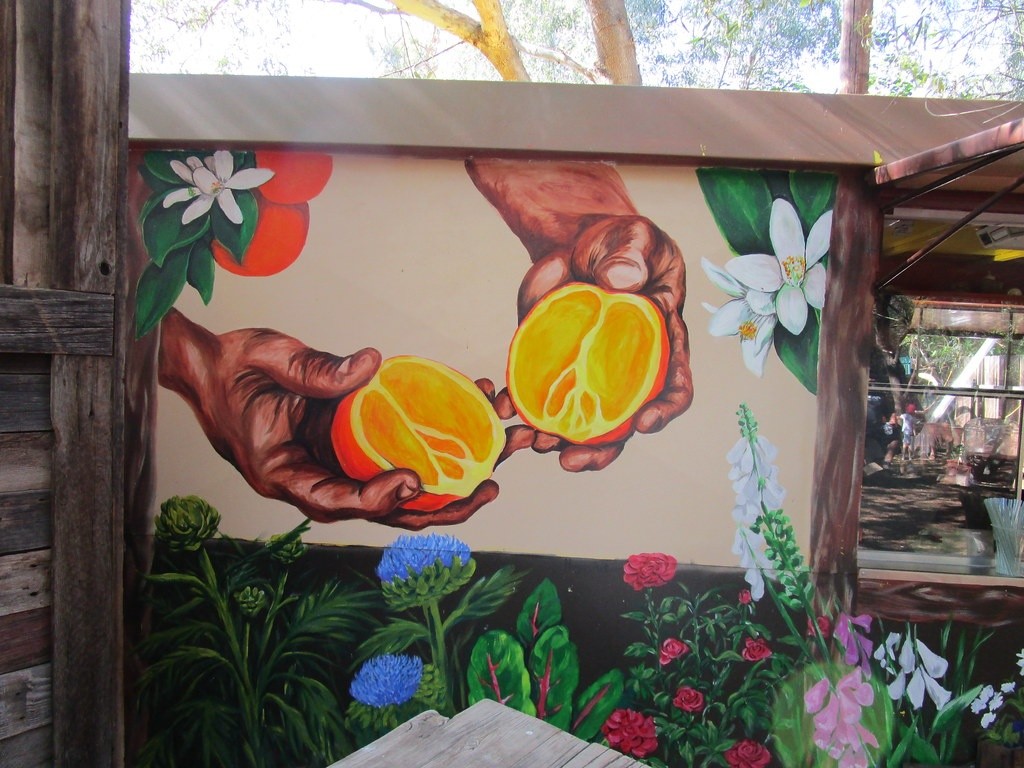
908;404;916;413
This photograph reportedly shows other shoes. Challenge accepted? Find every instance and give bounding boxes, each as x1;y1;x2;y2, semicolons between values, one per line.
909;458;912;460
901;457;905;460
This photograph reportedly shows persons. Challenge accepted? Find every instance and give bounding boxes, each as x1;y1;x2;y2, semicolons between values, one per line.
879;404;916;470
